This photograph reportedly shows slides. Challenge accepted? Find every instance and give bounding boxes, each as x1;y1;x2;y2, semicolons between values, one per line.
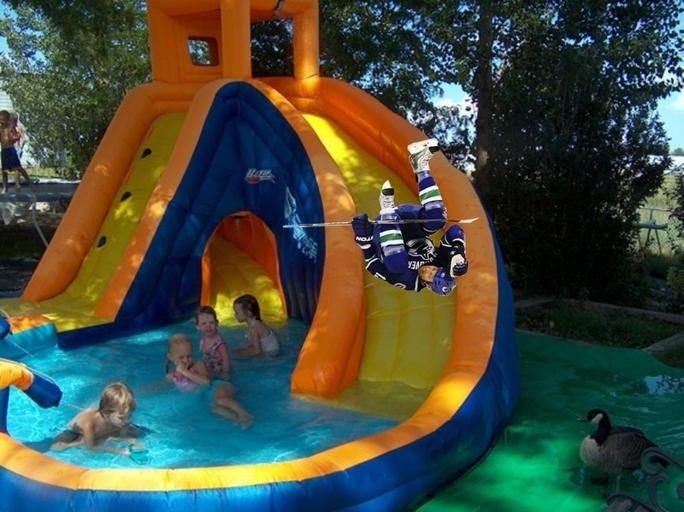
0;90;240;352
253;76;521;396
201;230;287;322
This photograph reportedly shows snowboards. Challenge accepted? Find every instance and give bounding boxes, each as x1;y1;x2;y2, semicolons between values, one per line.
281;220;478;229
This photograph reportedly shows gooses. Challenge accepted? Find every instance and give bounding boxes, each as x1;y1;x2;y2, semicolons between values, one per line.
576;408;671;495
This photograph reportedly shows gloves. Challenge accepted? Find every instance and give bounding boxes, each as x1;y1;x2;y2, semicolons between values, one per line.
351;214;374;250
450;246;468;276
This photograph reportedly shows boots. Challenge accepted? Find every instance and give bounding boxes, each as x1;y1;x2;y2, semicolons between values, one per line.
379;189;395;215
408;146;439;173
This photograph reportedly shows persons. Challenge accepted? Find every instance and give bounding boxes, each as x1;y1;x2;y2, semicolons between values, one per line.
351;137;467;297
50;382;144;458
230;293;280;360
193;305;231;381
8;113;27;191
164;330;256;431
0;110;36;196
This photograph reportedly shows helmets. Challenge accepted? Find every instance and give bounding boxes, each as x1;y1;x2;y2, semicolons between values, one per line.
432;267;457;296
